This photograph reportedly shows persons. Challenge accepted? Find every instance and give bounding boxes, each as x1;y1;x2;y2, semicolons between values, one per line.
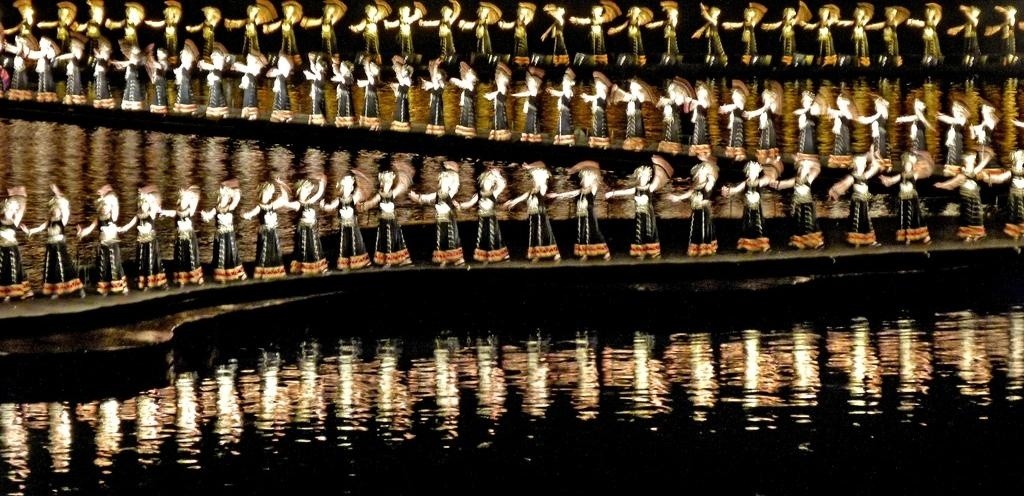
2;0;1024;175
1;161;612;308
604;145;1023;261
